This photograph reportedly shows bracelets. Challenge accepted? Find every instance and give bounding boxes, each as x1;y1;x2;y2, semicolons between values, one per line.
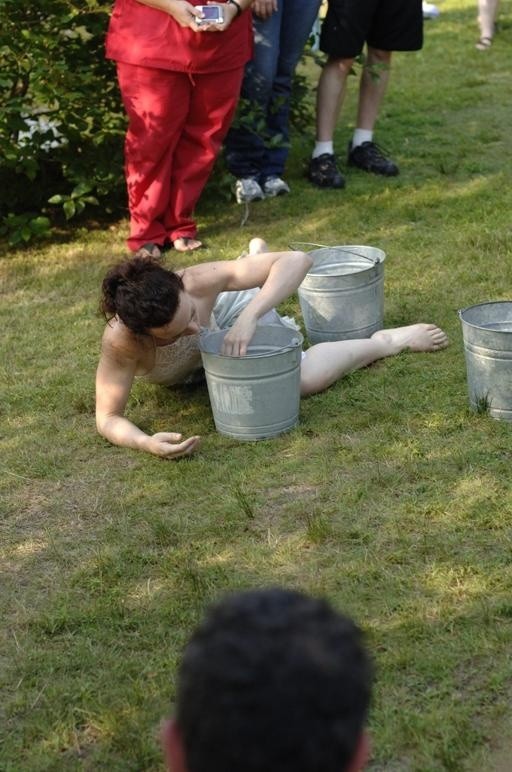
229;0;243;14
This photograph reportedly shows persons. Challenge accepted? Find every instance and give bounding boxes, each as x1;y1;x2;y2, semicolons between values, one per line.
157;588;375;772
474;0;500;50
221;1;324;207
105;1;259;265
90;234;451;460
305;0;426;188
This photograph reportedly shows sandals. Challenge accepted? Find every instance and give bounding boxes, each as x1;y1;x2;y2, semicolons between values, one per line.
476;36;492;50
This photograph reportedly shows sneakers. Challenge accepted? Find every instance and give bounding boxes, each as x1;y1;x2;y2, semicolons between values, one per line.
263;175;289;197
348;139;398;177
231;177;267;205
309;154;345;188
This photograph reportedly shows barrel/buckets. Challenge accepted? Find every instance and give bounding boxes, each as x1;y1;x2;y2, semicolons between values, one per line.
457;300;512;424
287;239;388;347
197;324;305;441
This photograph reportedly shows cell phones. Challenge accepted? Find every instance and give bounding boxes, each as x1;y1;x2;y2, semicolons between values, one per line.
196;5;223;24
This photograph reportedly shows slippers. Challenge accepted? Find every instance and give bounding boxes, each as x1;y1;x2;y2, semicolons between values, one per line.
134;242;161;262
175;238;204;251
